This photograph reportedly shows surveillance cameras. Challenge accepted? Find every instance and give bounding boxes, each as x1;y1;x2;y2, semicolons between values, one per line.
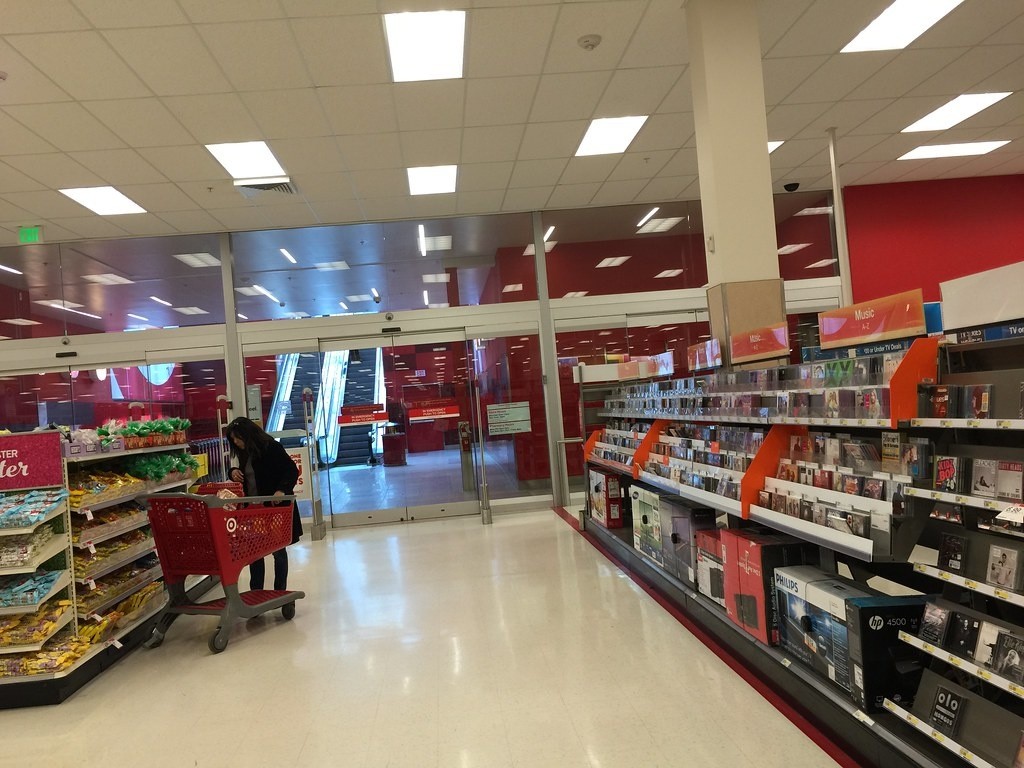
784;183;799;192
385;313;394;320
374;297;381;303
62;338;69;345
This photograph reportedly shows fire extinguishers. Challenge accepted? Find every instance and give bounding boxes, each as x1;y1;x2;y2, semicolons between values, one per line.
458;421;471;451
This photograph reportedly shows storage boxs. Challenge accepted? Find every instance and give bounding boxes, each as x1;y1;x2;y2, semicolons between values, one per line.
152;435;165;446
84;441;101;455
60;443;85;457
165;434;176;445
189;485;201;494
124;437;138;449
101;438;125;452
587;350;1024;740
190;453;209;478
139;436;153;448
175;431;184;444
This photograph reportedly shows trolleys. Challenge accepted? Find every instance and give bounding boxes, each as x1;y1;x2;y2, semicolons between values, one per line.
138;480;307;654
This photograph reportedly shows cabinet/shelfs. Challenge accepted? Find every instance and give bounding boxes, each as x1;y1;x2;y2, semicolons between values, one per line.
571;263;1024;768
0;429;222;709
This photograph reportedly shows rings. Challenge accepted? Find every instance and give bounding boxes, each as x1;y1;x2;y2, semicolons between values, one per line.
233;475;236;478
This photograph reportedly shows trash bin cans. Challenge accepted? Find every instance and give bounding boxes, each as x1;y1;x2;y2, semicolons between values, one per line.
381;422;407;467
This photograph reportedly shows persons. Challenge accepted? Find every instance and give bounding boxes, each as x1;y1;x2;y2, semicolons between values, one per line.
955;619;1021;677
226;416;303;620
979;476;992;488
995;552;1014;588
773;363;988;418
760;434;923;538
